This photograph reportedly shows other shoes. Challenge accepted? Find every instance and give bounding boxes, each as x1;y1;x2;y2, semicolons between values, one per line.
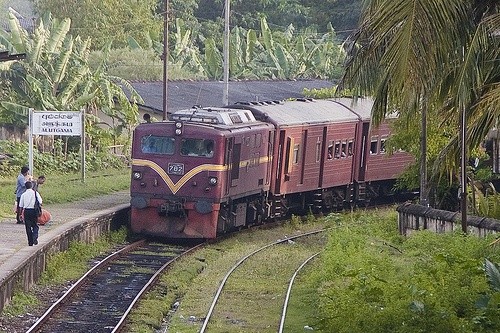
29;243;33;246
35;242;38;245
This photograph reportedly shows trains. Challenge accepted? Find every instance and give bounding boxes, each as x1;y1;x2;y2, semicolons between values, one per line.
130;96;495;241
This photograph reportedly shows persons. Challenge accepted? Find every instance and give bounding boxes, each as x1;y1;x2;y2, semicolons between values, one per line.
32;176;46;191
202;143;214;157
16;166;31;224
327;140;403;159
142;138;160;153
142;113;159;123
19;181;43;246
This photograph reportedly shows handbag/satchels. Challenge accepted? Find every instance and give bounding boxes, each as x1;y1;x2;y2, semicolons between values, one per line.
34;191;42;217
